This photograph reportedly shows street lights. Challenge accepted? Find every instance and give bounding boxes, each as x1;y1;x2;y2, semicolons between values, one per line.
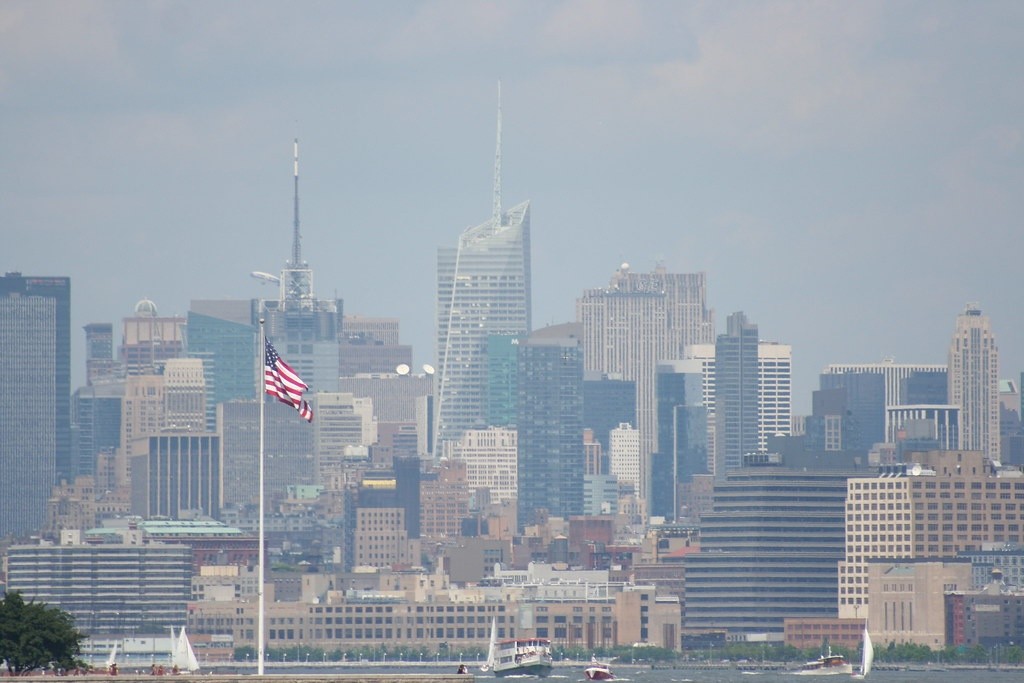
206;648;480;664
560;651;597;663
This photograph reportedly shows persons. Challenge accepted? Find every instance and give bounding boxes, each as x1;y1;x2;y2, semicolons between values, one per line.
457;664;468;674
36;661;180;676
517;651;552;660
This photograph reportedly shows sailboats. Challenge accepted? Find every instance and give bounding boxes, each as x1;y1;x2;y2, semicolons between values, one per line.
481;617;498;673
848;617;874;680
108;624;200;676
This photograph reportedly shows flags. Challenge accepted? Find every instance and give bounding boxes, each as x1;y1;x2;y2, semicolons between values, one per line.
264;336;315;423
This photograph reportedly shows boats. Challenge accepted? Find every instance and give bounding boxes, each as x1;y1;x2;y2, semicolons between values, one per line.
583;657;617;681
798;645;852;676
492;637;554;677
